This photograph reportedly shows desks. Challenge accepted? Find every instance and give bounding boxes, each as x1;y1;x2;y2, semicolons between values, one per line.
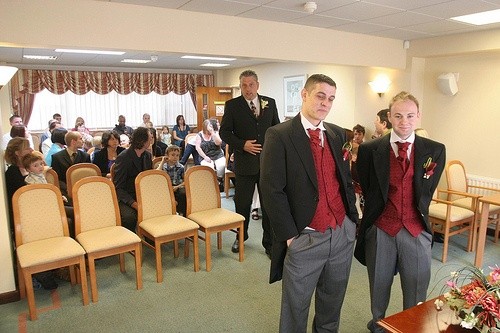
474;193;500;269
376;274;500;333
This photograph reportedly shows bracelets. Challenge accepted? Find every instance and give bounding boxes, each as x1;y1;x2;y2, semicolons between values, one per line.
178;183;185;192
129;199;135;207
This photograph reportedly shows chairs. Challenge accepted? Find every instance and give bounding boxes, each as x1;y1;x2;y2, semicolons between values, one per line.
427;178;475;263
445;161;499;244
71;177;144;303
11;183;88;322
46;170;61;188
184;166;247;272
135;169;199;283
65;163;102;198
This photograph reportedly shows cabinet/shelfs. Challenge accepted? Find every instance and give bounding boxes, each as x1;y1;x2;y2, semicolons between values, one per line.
196;86;233;132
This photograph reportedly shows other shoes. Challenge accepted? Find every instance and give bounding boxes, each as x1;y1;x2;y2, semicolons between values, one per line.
218;176;224;192
251;208;259;221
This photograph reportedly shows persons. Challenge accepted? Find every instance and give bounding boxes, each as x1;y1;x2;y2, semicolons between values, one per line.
351;124;365;183
219;69;281;259
4;113;262;289
375;109;392;136
259;73;359;333
355;90;448;333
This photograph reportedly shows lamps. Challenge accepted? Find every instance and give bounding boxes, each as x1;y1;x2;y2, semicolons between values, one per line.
368;81;390;99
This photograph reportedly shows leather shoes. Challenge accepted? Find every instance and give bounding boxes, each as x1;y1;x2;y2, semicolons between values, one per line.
231;239;239;253
264;249;271;260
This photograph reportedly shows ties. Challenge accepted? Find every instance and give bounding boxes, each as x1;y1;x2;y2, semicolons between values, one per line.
250;101;258;119
394;141;412;175
307;128;322;146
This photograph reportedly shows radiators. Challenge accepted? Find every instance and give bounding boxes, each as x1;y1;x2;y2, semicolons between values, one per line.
466;174;500;231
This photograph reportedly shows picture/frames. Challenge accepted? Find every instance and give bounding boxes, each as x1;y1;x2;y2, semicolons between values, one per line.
282;74;307;118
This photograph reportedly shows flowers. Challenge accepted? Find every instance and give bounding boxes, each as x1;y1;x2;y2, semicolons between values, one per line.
440;267;500;333
422;157;437;180
261;99;269;109
342;141;353;162
